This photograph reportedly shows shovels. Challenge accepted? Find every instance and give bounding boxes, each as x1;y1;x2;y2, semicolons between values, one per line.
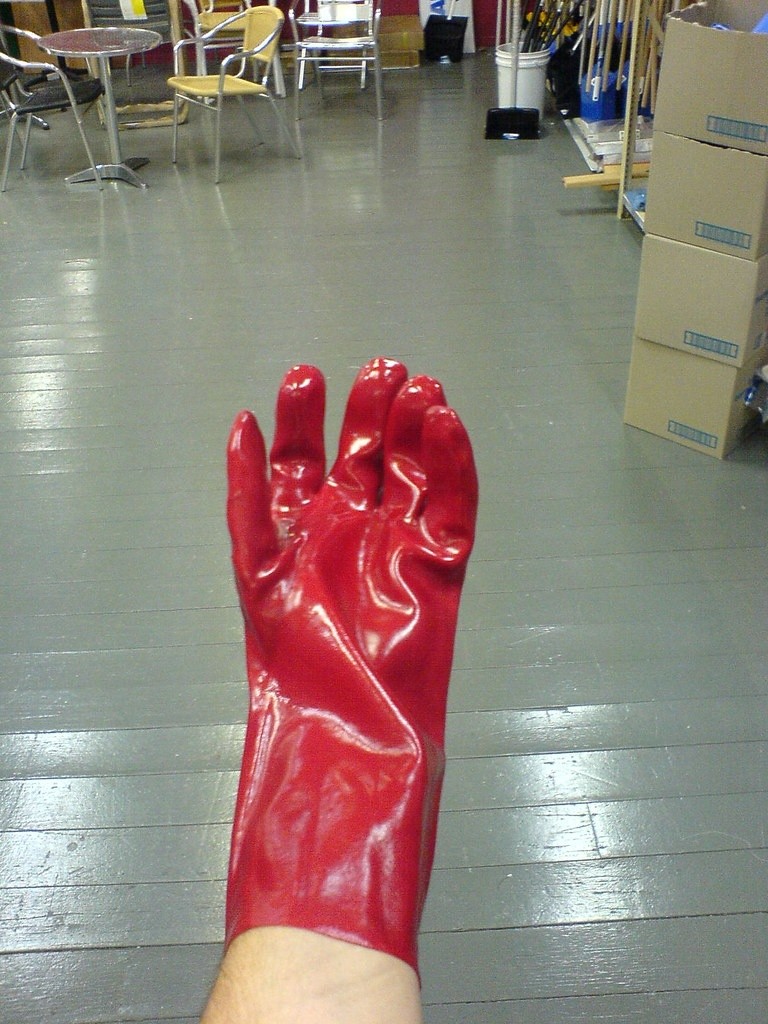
423;0;468;58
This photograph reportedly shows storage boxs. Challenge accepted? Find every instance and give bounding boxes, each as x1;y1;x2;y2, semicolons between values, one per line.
634;234;768;369
226;51;313;75
327;49;419;71
653;0;768;156
332;14;425;50
643;131;768;262
622;334;767;461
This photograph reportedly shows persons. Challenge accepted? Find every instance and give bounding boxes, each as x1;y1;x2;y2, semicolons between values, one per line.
197;355;482;1024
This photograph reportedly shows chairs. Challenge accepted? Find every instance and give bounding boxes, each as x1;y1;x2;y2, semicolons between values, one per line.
81;0;383;131
166;6;301;184
0;31;66;130
0;23;103;191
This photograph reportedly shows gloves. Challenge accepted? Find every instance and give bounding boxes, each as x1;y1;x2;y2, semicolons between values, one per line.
224;358;479;990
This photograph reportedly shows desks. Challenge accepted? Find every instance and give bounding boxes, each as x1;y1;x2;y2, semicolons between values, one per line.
37;27;163;190
